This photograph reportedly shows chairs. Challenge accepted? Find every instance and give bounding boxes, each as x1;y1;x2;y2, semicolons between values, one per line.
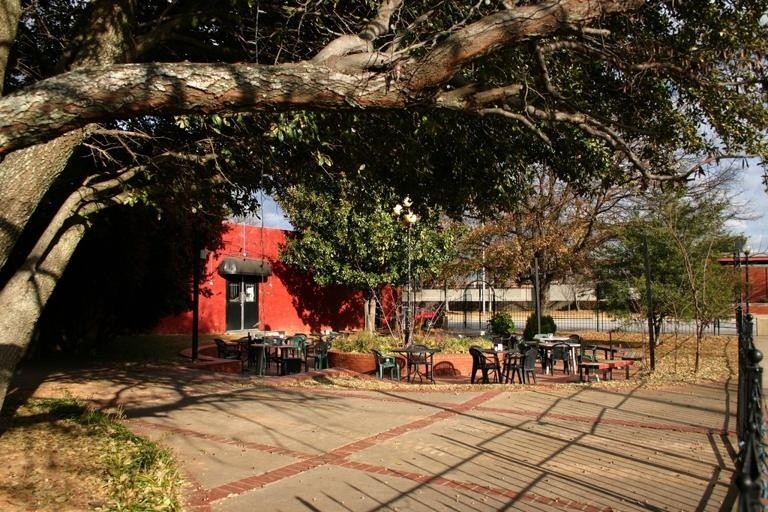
214;334;331;377
406;345;433;382
469;334;597;384
370;349;400;382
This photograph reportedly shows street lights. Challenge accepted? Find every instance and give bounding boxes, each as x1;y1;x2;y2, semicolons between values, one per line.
393;197;417;346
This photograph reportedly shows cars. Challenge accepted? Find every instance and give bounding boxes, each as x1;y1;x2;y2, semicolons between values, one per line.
403;306;443;329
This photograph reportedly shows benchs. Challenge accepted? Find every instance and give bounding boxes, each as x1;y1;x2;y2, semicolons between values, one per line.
578;360;633;382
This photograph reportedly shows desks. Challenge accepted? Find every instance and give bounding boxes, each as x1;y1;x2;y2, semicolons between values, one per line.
391;349;441;384
